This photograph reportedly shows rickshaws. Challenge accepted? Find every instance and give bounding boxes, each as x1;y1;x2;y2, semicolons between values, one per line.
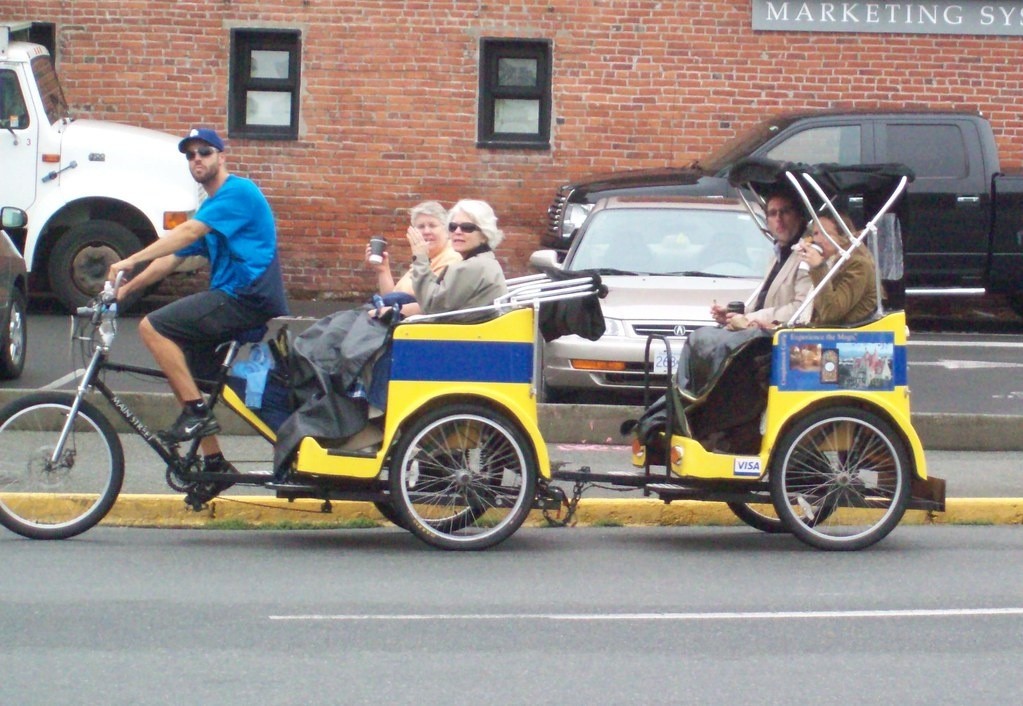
0;125;948;553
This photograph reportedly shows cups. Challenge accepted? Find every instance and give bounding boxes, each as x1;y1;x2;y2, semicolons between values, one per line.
726;301;745;314
798;244;824;271
368;235;388;264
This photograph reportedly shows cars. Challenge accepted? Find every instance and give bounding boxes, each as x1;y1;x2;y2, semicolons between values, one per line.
1;207;31;383
523;193;815;399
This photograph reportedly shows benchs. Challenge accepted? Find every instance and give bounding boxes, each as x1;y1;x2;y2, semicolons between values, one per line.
372;267;609;455
729;156;915;443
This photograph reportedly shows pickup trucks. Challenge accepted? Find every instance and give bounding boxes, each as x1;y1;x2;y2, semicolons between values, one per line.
540;109;1023;333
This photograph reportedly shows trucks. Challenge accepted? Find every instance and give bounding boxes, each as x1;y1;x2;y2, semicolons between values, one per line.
1;20;211;322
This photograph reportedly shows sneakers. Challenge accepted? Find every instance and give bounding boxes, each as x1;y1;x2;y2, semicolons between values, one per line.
156;404;221;441
184;458;240;504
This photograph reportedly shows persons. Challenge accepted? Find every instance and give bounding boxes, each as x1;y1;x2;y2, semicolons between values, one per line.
292;198;508;453
99;128;290;505
634;189;891;455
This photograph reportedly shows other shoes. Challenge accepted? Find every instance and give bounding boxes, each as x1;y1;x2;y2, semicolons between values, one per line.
338;421;383;451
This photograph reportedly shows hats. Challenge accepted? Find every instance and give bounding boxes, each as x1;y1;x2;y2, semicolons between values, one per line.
178;128;224;154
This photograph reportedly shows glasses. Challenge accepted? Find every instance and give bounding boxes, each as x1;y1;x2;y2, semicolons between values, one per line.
186;146;220;160
766;205;797;217
449;222;481;233
